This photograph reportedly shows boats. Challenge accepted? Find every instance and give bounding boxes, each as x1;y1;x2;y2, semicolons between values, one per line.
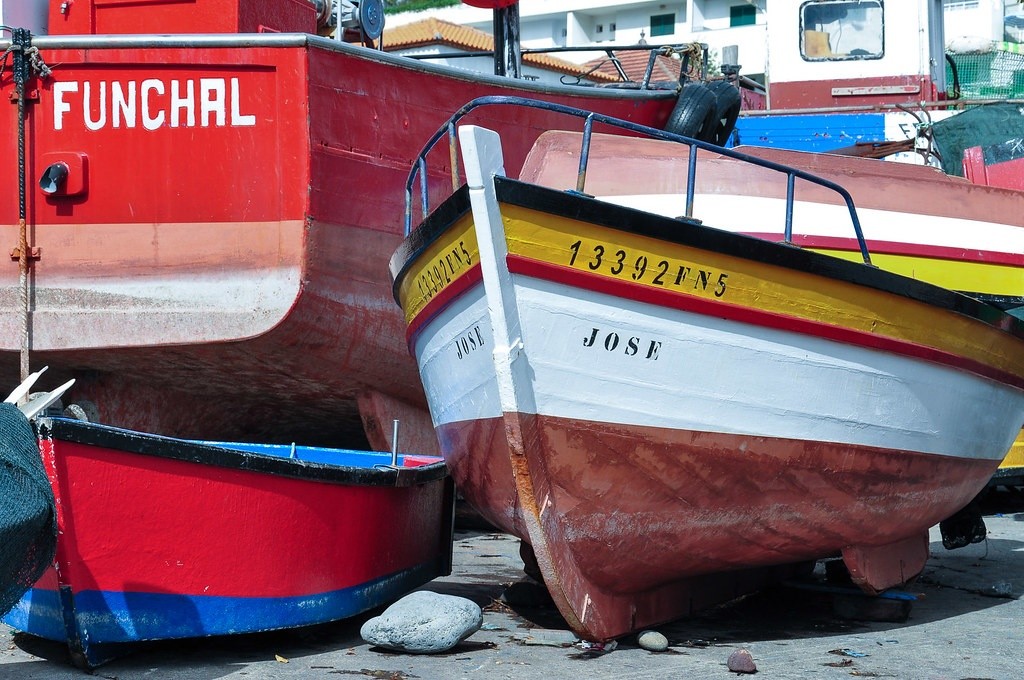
0;421;451;670
518;129;1023;484
716;0;1024;177
389;96;1024;641
5;0;739;526
